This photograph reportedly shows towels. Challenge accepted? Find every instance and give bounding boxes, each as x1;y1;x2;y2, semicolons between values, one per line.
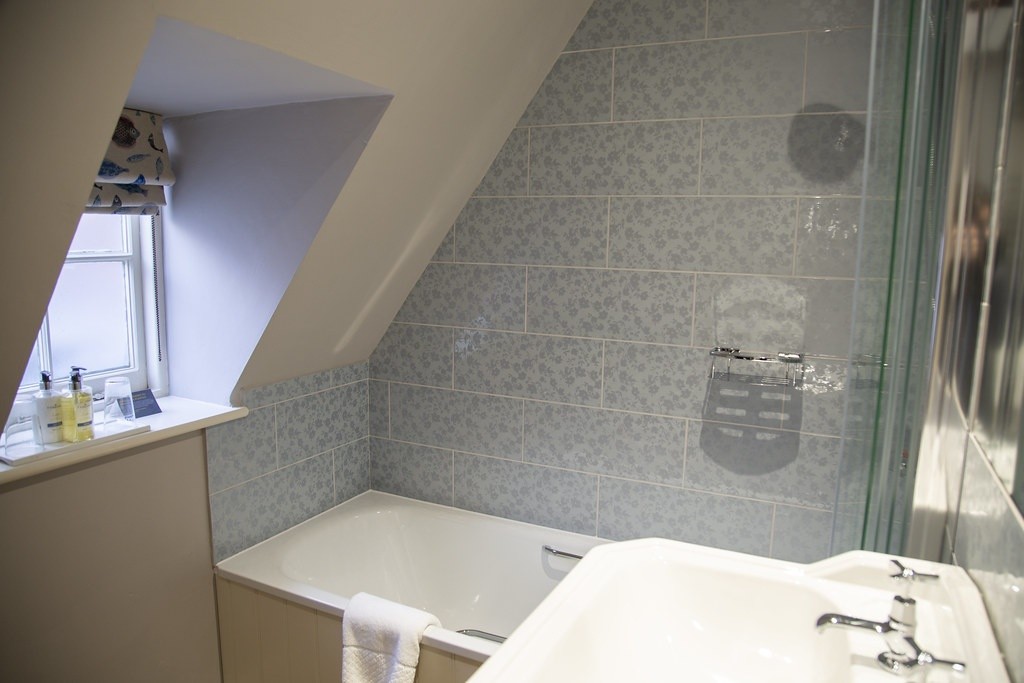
81;105;178;217
341;590;445;683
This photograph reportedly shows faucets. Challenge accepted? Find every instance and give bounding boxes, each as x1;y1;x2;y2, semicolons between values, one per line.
812;557;968;683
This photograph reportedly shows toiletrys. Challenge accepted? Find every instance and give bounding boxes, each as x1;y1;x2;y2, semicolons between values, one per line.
60;362;97;446
30;368;65;445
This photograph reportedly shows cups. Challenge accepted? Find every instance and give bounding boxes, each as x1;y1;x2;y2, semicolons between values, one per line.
4;394;46;458
102;375;136;434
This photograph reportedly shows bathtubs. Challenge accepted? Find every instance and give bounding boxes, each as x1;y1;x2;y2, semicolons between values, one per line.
215;486;622;683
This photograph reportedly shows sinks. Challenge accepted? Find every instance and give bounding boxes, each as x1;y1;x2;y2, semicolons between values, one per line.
459;537;1021;683
